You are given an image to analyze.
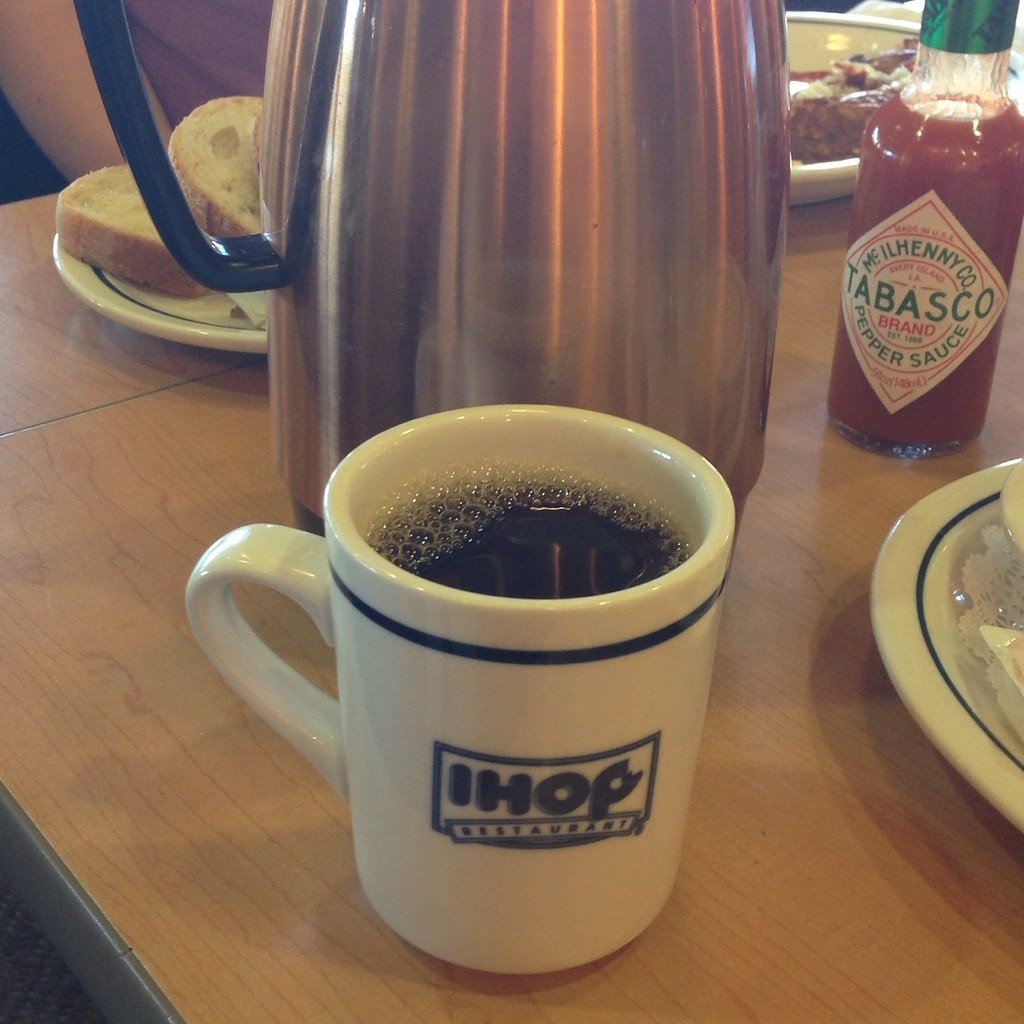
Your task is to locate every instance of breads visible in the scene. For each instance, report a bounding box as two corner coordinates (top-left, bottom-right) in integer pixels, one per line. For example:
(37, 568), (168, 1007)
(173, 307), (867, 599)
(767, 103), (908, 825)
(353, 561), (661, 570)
(56, 94), (263, 300)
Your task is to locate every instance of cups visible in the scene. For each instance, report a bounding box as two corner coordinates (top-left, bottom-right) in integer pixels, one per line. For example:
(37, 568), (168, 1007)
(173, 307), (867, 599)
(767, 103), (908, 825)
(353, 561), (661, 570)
(185, 403), (735, 974)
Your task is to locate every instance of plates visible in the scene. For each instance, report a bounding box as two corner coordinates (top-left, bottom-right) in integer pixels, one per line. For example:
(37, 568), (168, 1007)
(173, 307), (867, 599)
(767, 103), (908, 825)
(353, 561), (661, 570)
(784, 11), (1024, 209)
(868, 456), (1024, 836)
(48, 232), (266, 353)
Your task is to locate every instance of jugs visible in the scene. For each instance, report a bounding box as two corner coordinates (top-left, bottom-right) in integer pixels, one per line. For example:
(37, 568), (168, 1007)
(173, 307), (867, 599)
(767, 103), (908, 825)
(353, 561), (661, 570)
(74, 0), (789, 565)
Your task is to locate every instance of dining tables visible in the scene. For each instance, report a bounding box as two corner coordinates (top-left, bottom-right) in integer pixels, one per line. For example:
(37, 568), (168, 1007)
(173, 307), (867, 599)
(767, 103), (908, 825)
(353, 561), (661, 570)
(0, 0), (1024, 1023)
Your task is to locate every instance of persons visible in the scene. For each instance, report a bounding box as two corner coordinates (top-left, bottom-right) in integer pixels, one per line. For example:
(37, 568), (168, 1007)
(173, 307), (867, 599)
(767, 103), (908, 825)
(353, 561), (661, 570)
(0, 0), (273, 181)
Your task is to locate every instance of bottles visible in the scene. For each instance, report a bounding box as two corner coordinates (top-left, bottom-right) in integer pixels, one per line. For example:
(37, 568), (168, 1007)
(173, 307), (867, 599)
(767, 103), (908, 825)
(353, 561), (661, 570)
(827, 0), (1024, 457)
